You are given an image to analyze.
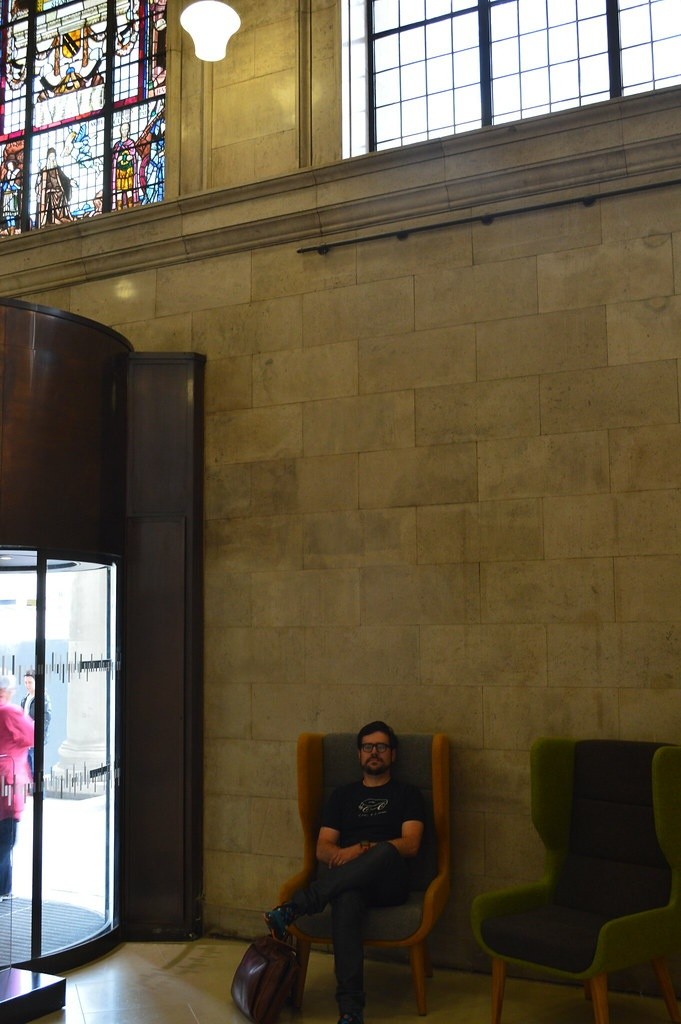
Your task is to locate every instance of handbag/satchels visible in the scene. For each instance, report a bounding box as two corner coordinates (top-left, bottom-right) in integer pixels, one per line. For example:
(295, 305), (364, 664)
(230, 927), (303, 1024)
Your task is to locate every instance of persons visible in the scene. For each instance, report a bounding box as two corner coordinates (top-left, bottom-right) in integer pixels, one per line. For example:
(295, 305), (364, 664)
(261, 721), (428, 1024)
(0, 675), (35, 902)
(19, 671), (52, 800)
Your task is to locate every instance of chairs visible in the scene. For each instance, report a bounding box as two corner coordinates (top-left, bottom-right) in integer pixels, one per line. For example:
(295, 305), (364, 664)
(468, 738), (680, 1024)
(277, 731), (451, 1016)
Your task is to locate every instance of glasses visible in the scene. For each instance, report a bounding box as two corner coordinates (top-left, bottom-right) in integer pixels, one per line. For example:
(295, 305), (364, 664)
(361, 743), (391, 753)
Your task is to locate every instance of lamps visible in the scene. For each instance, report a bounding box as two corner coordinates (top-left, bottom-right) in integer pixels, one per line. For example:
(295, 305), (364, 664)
(179, 0), (241, 62)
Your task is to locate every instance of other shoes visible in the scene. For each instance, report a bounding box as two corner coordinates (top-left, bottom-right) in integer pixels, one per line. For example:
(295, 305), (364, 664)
(0, 893), (16, 903)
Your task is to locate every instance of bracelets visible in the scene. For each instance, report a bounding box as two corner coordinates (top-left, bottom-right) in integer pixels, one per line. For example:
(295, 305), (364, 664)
(360, 841), (371, 852)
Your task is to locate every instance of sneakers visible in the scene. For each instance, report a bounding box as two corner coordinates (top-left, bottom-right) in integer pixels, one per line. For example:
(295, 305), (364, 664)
(262, 901), (299, 929)
(336, 1012), (364, 1024)
